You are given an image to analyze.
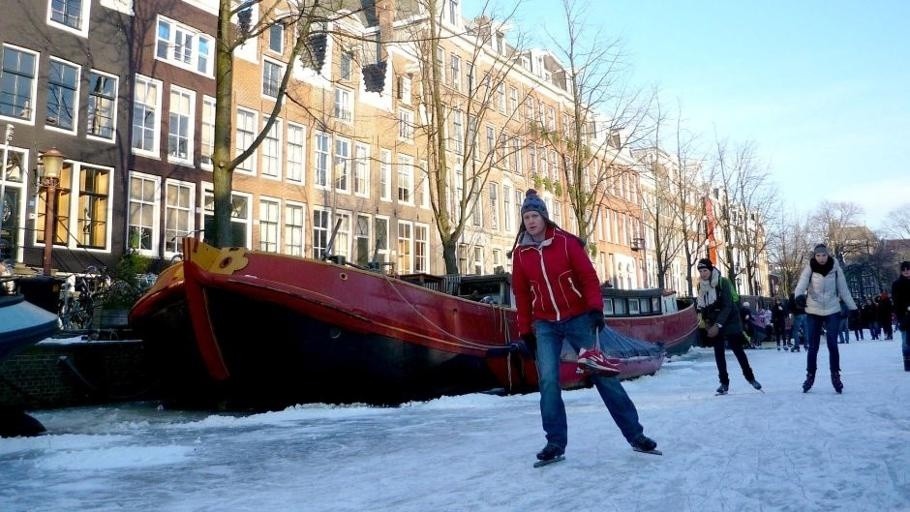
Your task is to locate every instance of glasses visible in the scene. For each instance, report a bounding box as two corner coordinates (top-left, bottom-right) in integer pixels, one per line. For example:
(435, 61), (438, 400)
(901, 267), (910, 271)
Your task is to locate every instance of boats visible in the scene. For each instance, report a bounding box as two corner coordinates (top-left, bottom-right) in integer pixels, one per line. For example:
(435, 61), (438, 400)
(127, 218), (664, 407)
(599, 279), (703, 359)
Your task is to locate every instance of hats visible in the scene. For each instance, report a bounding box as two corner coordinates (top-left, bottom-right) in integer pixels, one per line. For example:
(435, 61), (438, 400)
(697, 258), (713, 271)
(813, 243), (829, 257)
(520, 188), (549, 227)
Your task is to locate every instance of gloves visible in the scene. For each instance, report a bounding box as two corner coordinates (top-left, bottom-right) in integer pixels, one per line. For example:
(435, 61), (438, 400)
(521, 334), (538, 361)
(850, 309), (861, 321)
(590, 310), (605, 334)
(796, 295), (808, 308)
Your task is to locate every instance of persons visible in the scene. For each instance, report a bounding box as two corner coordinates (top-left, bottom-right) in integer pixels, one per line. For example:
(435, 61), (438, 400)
(696, 258), (762, 394)
(794, 242), (859, 391)
(504, 186), (658, 461)
(891, 260), (910, 372)
(692, 289), (893, 348)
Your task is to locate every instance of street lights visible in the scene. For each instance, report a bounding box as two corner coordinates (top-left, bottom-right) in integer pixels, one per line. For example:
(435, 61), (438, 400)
(37, 147), (72, 275)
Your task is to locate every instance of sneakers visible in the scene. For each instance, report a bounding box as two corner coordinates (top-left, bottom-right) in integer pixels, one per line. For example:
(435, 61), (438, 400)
(536, 444), (565, 461)
(716, 384), (728, 392)
(831, 375), (843, 388)
(631, 435), (657, 451)
(802, 374), (815, 389)
(576, 347), (623, 378)
(751, 380), (762, 390)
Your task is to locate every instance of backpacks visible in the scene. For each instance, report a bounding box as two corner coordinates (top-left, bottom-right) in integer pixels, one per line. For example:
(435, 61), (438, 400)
(718, 276), (740, 304)
